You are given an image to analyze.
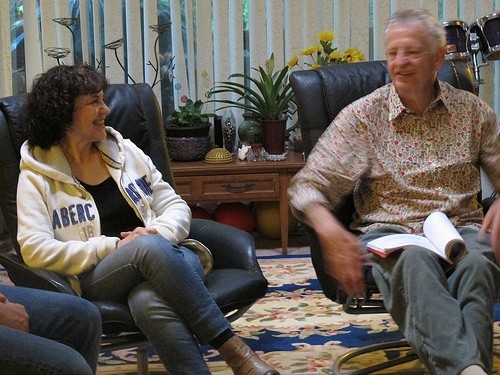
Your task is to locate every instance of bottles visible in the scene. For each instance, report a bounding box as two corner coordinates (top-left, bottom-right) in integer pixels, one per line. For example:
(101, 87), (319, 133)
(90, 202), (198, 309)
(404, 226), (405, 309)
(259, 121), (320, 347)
(238, 110), (262, 146)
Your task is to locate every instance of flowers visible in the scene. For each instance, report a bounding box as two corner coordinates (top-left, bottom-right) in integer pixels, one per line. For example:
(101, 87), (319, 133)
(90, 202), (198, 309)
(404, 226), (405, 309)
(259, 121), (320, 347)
(287, 29), (367, 70)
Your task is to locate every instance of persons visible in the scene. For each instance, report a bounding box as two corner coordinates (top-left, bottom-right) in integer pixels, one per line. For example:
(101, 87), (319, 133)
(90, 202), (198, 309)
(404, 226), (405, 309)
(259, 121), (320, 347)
(16, 65), (280, 375)
(286, 10), (500, 375)
(0, 284), (102, 375)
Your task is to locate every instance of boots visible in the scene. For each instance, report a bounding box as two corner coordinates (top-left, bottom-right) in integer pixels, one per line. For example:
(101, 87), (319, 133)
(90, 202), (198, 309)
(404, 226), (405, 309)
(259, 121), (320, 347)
(216, 334), (280, 375)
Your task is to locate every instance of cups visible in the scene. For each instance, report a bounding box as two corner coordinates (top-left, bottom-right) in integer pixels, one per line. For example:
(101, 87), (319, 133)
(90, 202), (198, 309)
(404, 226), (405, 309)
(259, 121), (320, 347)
(247, 148), (260, 160)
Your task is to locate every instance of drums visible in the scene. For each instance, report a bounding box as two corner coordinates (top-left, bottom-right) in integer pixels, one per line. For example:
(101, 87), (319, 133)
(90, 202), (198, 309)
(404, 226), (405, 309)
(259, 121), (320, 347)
(441, 19), (471, 62)
(471, 10), (500, 60)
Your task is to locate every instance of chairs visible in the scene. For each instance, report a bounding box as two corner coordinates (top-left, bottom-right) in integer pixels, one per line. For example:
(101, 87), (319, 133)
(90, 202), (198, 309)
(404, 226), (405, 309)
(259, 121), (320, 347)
(290, 58), (460, 375)
(0, 82), (270, 375)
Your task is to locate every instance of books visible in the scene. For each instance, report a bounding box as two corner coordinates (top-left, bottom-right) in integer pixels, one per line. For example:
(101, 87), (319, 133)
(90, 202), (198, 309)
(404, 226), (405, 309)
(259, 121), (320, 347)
(366, 212), (467, 273)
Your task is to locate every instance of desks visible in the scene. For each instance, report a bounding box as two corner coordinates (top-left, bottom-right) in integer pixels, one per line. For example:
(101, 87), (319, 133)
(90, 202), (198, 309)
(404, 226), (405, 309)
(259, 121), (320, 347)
(170, 147), (306, 256)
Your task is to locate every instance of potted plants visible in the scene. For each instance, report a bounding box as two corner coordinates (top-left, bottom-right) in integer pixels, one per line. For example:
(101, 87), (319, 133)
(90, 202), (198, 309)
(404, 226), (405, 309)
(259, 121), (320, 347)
(203, 52), (297, 155)
(162, 98), (212, 163)
